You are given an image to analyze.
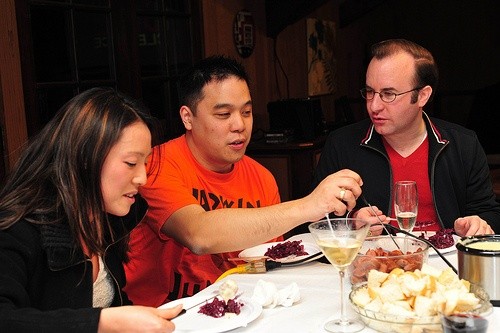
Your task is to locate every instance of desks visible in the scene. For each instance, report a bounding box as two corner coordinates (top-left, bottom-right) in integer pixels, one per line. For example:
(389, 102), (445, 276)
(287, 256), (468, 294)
(197, 233), (500, 333)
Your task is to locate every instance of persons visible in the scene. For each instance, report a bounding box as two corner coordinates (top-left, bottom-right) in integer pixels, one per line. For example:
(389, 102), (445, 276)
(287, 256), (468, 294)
(121, 55), (364, 308)
(0, 87), (184, 333)
(310, 40), (500, 240)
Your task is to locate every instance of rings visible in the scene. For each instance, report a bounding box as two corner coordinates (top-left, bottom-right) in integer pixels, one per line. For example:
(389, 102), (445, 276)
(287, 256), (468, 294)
(367, 231), (372, 237)
(339, 188), (346, 200)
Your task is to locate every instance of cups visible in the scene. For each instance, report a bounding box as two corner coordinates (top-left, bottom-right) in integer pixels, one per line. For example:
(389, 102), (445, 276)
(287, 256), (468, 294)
(438, 301), (494, 333)
(457, 235), (500, 307)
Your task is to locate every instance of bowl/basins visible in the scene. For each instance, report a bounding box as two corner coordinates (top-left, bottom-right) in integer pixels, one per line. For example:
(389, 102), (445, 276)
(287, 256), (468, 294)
(349, 282), (490, 333)
(348, 236), (428, 286)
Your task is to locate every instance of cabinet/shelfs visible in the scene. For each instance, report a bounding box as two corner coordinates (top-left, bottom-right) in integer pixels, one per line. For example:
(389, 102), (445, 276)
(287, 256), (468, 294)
(246, 139), (325, 203)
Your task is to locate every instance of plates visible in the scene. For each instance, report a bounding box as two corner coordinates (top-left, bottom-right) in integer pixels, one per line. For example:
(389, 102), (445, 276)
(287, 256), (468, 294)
(379, 231), (461, 256)
(239, 242), (324, 265)
(156, 296), (260, 333)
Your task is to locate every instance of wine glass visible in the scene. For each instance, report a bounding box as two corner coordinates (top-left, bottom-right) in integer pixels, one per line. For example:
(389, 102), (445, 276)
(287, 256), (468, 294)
(307, 218), (374, 333)
(394, 180), (417, 252)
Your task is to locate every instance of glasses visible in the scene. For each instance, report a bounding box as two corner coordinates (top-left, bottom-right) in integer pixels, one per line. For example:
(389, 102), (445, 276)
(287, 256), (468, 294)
(360, 85), (420, 105)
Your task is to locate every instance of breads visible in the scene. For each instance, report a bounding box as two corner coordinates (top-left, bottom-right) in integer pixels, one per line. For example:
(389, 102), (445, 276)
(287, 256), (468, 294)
(353, 266), (481, 317)
